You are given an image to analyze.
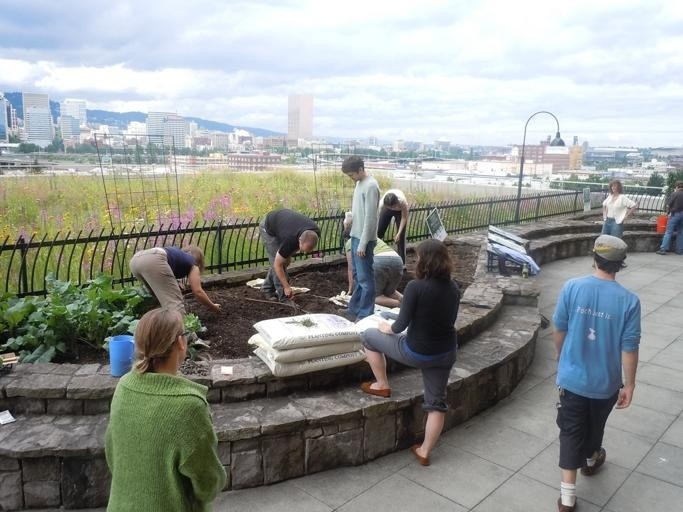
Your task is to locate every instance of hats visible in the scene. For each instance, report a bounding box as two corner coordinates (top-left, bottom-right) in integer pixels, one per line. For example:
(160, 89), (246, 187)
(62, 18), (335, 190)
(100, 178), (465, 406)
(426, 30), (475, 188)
(592, 235), (626, 261)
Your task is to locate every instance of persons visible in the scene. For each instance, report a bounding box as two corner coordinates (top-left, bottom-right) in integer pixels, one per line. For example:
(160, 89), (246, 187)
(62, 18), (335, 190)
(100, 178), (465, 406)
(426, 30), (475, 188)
(102, 307), (228, 511)
(378, 189), (408, 271)
(551, 232), (641, 512)
(601, 180), (637, 268)
(256, 208), (321, 300)
(128, 243), (222, 321)
(655, 180), (683, 255)
(342, 210), (404, 307)
(338, 155), (381, 321)
(356, 238), (460, 468)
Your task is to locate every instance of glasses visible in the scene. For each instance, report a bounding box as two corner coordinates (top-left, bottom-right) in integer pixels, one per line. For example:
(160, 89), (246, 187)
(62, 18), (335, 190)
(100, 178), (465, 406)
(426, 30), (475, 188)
(181, 330), (189, 336)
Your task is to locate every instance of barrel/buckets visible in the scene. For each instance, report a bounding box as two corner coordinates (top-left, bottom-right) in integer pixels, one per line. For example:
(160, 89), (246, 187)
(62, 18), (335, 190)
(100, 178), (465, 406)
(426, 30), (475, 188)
(108, 335), (135, 378)
(656, 215), (668, 234)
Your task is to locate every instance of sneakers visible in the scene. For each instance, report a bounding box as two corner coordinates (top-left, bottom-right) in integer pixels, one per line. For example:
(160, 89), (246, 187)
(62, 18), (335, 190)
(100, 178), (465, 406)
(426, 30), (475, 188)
(557, 496), (576, 512)
(262, 292), (277, 302)
(338, 309), (355, 322)
(360, 383), (390, 397)
(655, 250), (665, 255)
(580, 448), (605, 476)
(411, 445), (429, 466)
(282, 300), (300, 308)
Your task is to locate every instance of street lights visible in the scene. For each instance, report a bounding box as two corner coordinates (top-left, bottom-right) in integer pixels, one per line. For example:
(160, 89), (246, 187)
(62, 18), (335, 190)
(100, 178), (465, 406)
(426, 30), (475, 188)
(513, 110), (566, 224)
